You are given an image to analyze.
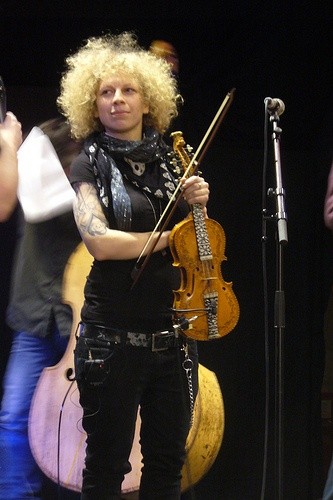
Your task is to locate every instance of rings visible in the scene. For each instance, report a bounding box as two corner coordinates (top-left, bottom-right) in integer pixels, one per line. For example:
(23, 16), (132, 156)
(198, 183), (202, 190)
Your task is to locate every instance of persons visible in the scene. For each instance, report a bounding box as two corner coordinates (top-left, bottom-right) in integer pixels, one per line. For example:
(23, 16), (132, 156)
(0, 111), (23, 222)
(58, 38), (210, 500)
(1, 113), (85, 500)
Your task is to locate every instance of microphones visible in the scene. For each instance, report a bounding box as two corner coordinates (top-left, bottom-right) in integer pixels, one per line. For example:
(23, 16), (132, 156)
(263, 96), (286, 116)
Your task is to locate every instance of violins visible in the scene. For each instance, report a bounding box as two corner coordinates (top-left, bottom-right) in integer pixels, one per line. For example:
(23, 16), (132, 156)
(170, 132), (240, 340)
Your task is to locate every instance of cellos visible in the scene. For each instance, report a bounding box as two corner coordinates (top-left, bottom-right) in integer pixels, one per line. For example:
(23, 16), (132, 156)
(27, 42), (225, 500)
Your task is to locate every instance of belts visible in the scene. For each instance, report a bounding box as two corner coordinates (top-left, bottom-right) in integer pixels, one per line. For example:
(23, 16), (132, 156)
(80, 323), (188, 352)
